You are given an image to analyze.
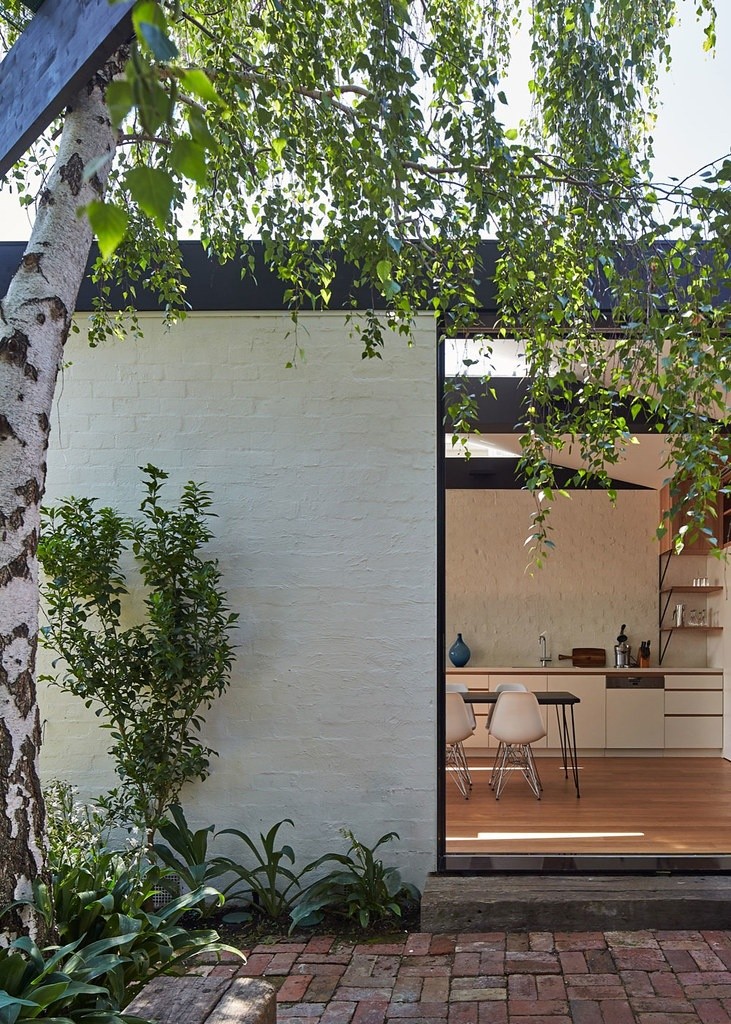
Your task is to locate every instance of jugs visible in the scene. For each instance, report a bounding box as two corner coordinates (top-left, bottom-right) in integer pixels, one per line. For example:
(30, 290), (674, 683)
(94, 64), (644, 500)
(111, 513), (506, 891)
(672, 603), (688, 627)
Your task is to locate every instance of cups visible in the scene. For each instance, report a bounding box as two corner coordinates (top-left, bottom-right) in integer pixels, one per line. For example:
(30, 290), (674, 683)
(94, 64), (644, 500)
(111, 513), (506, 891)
(614, 645), (632, 668)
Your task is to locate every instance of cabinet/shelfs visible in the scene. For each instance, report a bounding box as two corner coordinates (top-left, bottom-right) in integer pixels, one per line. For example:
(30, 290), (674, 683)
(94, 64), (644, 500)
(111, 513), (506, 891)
(664, 668), (723, 757)
(659, 479), (725, 555)
(658, 550), (724, 667)
(445, 667), (488, 758)
(488, 668), (605, 757)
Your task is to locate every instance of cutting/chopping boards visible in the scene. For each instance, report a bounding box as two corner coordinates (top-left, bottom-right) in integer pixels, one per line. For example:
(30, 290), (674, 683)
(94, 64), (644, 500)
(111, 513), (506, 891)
(558, 647), (606, 668)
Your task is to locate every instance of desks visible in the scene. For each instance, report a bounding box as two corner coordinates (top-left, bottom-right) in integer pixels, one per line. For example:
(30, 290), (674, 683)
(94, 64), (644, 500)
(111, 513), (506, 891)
(458, 691), (582, 799)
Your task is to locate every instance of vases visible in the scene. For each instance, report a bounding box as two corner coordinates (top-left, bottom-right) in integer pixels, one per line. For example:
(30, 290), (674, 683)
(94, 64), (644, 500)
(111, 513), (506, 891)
(448, 633), (471, 668)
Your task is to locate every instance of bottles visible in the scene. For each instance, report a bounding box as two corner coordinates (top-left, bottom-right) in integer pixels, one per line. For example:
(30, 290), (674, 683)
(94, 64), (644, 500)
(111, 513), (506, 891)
(449, 632), (471, 667)
(689, 609), (708, 626)
(692, 575), (709, 587)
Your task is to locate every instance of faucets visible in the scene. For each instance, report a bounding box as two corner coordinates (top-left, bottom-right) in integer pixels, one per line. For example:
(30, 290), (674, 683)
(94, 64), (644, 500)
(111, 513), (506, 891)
(537, 635), (553, 667)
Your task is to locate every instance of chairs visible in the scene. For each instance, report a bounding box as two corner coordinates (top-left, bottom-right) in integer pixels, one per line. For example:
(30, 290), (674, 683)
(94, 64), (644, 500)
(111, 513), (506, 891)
(486, 682), (544, 793)
(446, 682), (477, 787)
(446, 691), (476, 800)
(489, 691), (548, 801)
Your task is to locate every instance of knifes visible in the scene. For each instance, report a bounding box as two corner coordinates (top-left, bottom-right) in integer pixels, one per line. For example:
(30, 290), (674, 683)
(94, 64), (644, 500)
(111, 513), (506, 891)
(640, 640), (651, 656)
